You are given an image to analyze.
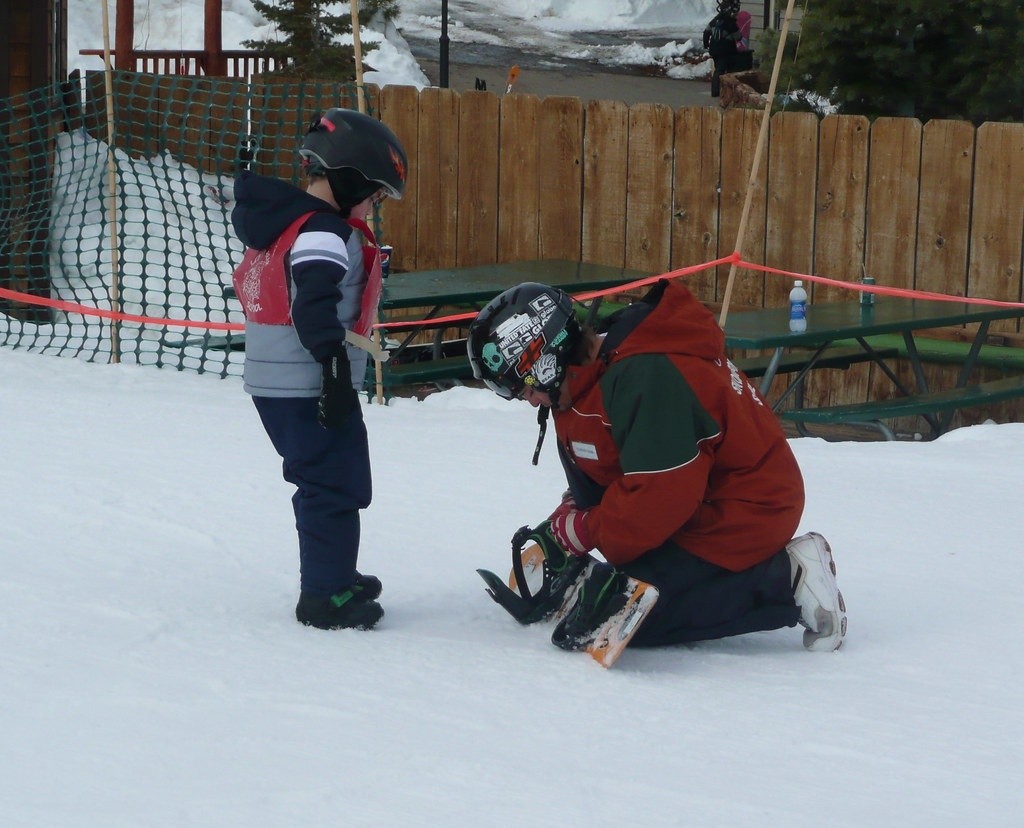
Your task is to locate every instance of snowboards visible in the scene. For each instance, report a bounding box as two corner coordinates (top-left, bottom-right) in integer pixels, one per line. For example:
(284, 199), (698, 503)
(507, 543), (659, 668)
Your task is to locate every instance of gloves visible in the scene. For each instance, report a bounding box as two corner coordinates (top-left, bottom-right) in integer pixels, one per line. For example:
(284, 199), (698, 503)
(547, 494), (578, 522)
(550, 511), (592, 558)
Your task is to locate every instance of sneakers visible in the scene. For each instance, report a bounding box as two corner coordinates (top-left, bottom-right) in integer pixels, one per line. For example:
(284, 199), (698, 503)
(784, 531), (848, 653)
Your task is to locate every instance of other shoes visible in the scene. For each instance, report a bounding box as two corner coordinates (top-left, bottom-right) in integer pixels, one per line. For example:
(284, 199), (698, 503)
(355, 572), (382, 601)
(295, 592), (385, 630)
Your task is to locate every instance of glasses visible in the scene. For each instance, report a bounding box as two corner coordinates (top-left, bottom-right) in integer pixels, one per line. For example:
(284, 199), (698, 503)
(369, 188), (388, 205)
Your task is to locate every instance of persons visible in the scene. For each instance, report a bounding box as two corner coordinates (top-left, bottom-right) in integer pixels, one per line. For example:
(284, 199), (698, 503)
(230, 108), (409, 632)
(466, 284), (848, 671)
(703, 0), (743, 98)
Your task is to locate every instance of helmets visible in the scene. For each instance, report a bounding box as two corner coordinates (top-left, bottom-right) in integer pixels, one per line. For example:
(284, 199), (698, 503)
(465, 281), (574, 401)
(298, 107), (408, 200)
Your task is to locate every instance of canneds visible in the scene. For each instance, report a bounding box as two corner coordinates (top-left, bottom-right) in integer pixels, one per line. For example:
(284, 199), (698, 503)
(859, 277), (874, 305)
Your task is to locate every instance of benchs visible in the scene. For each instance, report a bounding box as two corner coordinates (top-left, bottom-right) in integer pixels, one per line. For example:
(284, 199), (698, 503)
(726, 345), (899, 408)
(370, 357), (473, 392)
(781, 376), (1024, 441)
(388, 310), (473, 335)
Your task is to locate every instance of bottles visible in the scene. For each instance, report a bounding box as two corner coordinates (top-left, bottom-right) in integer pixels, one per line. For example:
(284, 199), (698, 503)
(790, 281), (807, 331)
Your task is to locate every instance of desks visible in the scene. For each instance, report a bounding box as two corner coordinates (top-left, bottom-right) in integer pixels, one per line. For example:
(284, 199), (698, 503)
(383, 258), (658, 366)
(717, 295), (1024, 438)
